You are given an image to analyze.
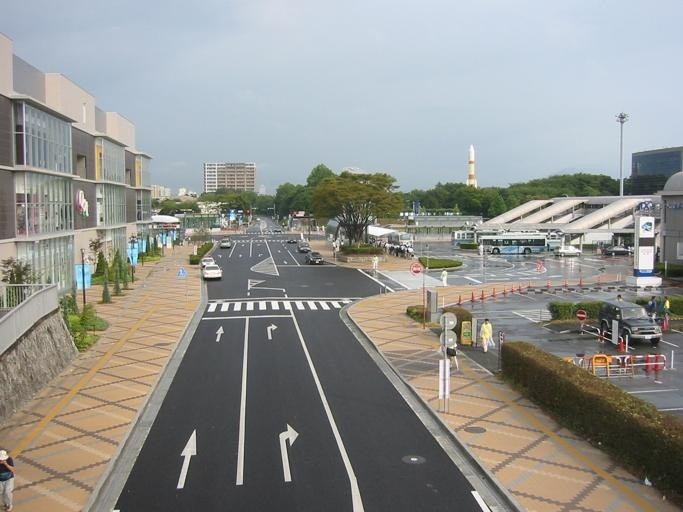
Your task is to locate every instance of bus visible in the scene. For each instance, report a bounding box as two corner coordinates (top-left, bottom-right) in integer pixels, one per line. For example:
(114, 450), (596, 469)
(452, 229), (561, 249)
(477, 234), (548, 255)
(387, 232), (413, 249)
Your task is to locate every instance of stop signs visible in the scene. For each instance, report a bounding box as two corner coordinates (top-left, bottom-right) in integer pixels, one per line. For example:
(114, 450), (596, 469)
(576, 310), (586, 321)
(410, 262), (424, 276)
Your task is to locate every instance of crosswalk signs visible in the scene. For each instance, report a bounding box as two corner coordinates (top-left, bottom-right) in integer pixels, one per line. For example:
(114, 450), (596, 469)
(177, 267), (186, 277)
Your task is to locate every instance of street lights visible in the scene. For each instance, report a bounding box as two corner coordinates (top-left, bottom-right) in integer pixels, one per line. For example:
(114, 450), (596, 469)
(125, 233), (137, 285)
(79, 248), (87, 306)
(615, 110), (630, 196)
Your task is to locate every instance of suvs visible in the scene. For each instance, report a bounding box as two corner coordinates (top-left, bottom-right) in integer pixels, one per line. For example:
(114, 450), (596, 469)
(599, 300), (661, 347)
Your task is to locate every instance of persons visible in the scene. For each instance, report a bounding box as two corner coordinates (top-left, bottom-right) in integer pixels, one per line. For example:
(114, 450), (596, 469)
(614, 295), (670, 321)
(445, 342), (458, 371)
(439, 269), (447, 287)
(478, 319), (492, 352)
(331, 236), (414, 260)
(0, 449), (15, 511)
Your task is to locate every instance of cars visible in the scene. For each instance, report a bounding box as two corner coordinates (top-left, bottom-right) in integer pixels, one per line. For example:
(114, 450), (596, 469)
(304, 251), (323, 265)
(202, 256), (215, 268)
(604, 245), (629, 255)
(298, 240), (311, 252)
(287, 236), (297, 244)
(273, 226), (281, 232)
(202, 264), (222, 279)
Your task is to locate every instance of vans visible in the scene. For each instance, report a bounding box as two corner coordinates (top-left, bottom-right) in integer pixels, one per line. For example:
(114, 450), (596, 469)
(553, 245), (581, 258)
(220, 238), (231, 249)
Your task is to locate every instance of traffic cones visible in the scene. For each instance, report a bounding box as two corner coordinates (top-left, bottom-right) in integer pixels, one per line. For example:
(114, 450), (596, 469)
(660, 318), (667, 331)
(618, 338), (625, 352)
(645, 353), (653, 372)
(654, 354), (662, 371)
